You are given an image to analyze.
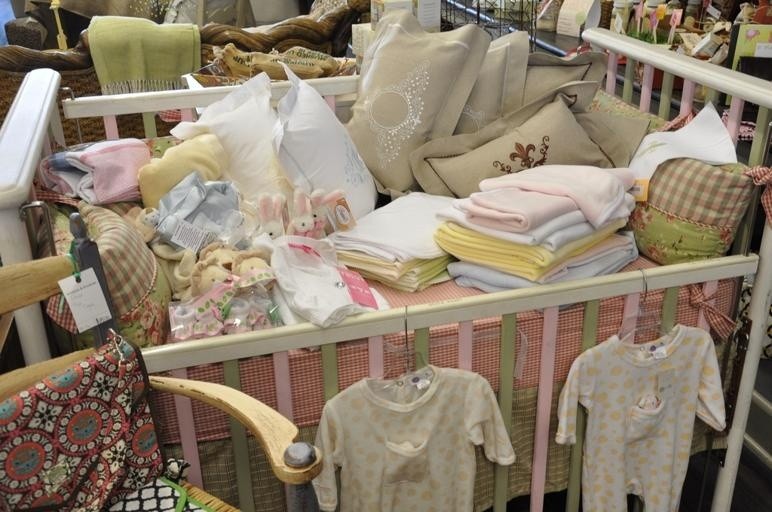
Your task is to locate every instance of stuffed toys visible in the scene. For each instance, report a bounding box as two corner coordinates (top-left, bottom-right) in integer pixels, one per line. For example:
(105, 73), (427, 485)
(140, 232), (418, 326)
(286, 189), (315, 238)
(259, 196), (284, 241)
(124, 206), (188, 262)
(311, 188), (345, 241)
(191, 243), (277, 301)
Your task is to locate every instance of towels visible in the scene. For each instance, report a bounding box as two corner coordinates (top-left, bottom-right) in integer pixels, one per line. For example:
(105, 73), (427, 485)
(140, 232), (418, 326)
(433, 163), (639, 289)
(324, 191), (473, 293)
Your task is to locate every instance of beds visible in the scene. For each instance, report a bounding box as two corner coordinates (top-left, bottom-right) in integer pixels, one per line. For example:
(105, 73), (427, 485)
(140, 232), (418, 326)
(0, 23), (772, 512)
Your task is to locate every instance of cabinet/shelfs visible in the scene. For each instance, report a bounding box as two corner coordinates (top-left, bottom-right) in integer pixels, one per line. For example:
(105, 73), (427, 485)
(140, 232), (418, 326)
(440, 0), (772, 168)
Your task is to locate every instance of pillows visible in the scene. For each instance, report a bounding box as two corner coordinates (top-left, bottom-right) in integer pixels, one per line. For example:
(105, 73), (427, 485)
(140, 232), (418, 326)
(35, 202), (95, 352)
(449, 24), (531, 142)
(623, 162), (756, 265)
(342, 9), (492, 202)
(519, 46), (608, 119)
(74, 192), (171, 354)
(407, 80), (630, 220)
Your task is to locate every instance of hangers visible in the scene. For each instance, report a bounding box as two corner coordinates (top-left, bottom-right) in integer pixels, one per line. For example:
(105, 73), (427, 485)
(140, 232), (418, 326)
(366, 304), (436, 398)
(614, 269), (676, 345)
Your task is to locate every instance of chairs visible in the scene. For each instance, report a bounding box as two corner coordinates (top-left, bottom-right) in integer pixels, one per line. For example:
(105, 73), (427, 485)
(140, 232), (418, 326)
(0, 235), (324, 512)
